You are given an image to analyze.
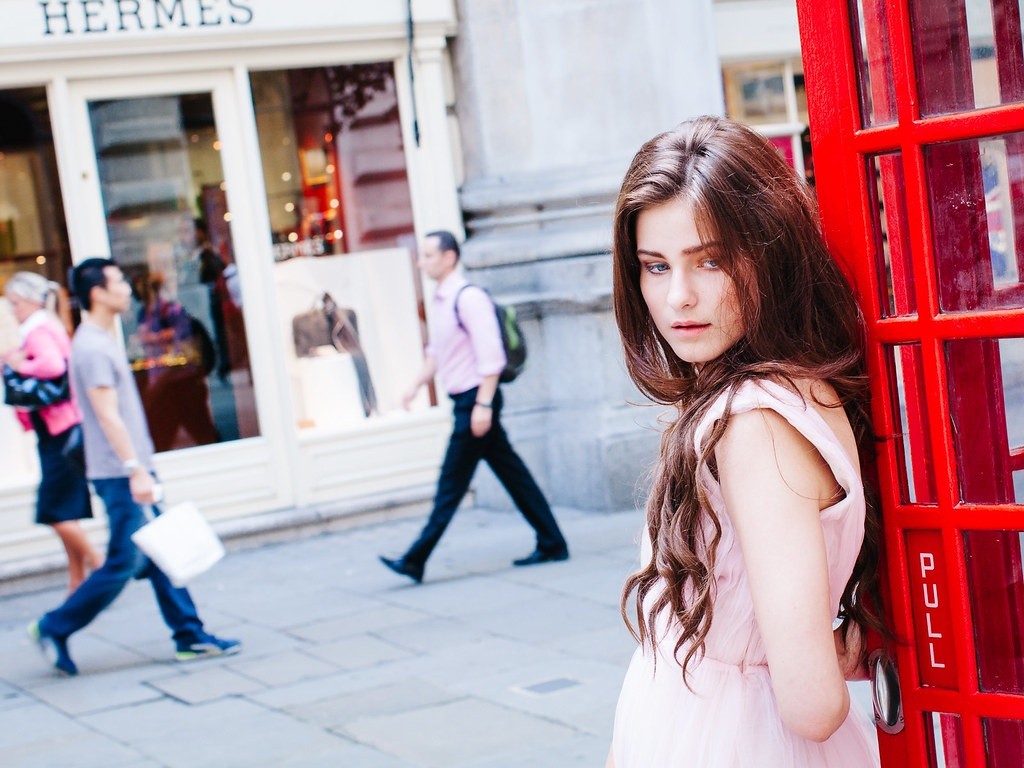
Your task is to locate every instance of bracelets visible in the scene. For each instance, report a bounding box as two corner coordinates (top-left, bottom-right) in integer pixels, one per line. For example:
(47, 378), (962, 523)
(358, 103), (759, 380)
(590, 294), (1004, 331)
(475, 401), (492, 408)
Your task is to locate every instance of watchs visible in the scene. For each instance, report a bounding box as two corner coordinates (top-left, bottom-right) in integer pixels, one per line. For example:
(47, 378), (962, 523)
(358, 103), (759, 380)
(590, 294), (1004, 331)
(121, 459), (140, 476)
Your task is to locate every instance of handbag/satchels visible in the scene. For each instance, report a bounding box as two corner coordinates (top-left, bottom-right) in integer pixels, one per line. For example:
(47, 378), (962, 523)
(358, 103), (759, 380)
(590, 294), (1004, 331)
(131, 498), (226, 588)
(3, 341), (71, 406)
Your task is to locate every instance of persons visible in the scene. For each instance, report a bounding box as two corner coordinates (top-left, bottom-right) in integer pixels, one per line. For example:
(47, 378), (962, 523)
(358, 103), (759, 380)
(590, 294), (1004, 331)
(0, 271), (104, 605)
(115, 262), (230, 453)
(602, 112), (911, 768)
(24, 258), (241, 678)
(378, 230), (570, 584)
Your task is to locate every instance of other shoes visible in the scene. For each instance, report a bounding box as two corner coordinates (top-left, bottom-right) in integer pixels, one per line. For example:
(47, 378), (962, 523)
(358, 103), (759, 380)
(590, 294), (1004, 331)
(513, 550), (569, 565)
(379, 557), (422, 582)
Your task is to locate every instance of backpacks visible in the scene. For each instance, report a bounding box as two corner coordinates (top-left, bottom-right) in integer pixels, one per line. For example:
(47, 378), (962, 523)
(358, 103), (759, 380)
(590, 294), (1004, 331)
(158, 302), (216, 383)
(454, 284), (528, 383)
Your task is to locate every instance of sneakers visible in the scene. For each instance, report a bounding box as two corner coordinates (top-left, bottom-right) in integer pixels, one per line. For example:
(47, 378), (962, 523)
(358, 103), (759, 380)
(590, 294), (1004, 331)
(175, 636), (241, 661)
(28, 620), (78, 677)
